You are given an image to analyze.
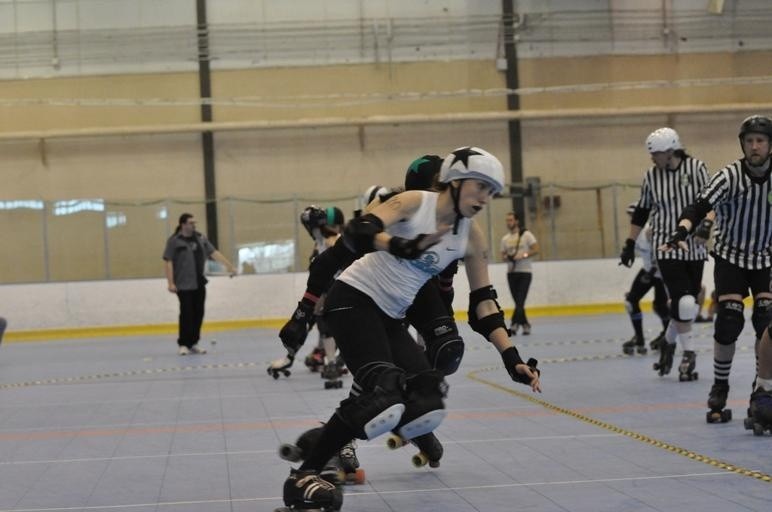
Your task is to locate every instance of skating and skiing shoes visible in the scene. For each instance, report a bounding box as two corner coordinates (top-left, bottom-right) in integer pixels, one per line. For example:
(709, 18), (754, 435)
(678, 350), (699, 381)
(623, 335), (647, 355)
(706, 384), (731, 423)
(386, 429), (443, 467)
(267, 347), (348, 388)
(744, 375), (772, 435)
(278, 421), (366, 512)
(650, 331), (677, 376)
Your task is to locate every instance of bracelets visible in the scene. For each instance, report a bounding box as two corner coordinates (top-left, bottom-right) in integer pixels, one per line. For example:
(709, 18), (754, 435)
(523, 252), (528, 258)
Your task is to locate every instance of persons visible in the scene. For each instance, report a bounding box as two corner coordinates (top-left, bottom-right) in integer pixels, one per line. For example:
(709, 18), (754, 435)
(500, 213), (539, 335)
(624, 201), (672, 347)
(283, 155), (465, 485)
(276, 186), (388, 390)
(282, 147), (543, 511)
(619, 126), (716, 373)
(162, 213), (239, 355)
(655, 112), (772, 424)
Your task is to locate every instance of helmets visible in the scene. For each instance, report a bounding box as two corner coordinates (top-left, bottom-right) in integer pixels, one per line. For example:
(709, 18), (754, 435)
(405, 155), (442, 191)
(364, 185), (388, 204)
(738, 115), (772, 139)
(645, 128), (686, 153)
(321, 206), (343, 228)
(437, 146), (506, 192)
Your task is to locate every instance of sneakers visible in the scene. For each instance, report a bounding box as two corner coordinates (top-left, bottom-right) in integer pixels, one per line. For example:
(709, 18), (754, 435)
(511, 322), (519, 336)
(522, 323), (531, 334)
(178, 345), (206, 355)
